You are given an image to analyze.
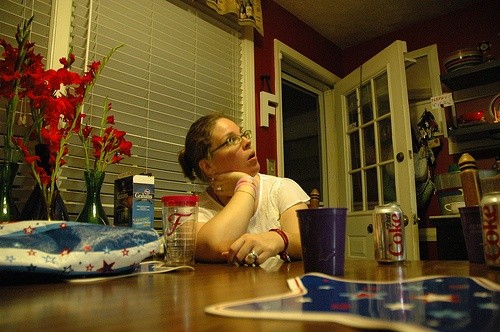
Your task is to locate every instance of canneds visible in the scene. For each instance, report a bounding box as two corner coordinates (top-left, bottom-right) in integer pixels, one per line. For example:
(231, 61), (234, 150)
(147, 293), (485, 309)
(372, 204), (406, 265)
(480, 191), (500, 267)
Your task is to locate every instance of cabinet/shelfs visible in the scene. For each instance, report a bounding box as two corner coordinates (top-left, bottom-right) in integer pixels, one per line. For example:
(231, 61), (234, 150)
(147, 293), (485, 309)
(430, 215), (464, 225)
(439, 62), (500, 156)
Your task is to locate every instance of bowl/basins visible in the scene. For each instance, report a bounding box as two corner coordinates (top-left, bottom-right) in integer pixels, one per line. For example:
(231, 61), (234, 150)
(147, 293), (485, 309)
(443, 48), (482, 74)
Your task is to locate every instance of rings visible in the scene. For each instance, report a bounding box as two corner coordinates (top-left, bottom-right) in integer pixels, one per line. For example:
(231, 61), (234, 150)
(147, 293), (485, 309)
(250, 249), (259, 260)
(216, 185), (223, 192)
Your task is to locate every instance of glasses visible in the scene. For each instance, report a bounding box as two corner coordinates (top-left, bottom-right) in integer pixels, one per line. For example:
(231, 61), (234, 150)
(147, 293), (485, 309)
(209, 130), (252, 154)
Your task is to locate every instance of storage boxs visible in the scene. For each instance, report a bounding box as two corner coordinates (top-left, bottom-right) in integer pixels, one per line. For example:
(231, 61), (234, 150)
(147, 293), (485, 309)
(114, 175), (154, 230)
(433, 169), (499, 216)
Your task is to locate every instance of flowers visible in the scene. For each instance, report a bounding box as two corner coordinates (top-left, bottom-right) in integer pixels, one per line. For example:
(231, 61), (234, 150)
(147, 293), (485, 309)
(0, 15), (66, 162)
(10, 43), (124, 144)
(13, 133), (73, 190)
(76, 95), (133, 173)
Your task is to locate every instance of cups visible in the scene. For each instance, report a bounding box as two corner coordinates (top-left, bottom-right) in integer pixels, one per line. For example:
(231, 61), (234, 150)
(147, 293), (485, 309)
(444, 202), (466, 215)
(296, 208), (347, 277)
(161, 195), (199, 267)
(459, 206), (485, 264)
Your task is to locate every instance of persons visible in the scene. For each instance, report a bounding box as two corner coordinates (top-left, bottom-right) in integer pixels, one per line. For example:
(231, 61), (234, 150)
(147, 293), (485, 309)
(176, 111), (311, 268)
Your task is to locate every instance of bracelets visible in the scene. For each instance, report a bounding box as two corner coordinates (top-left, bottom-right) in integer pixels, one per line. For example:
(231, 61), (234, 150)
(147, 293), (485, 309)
(234, 179), (258, 201)
(267, 228), (292, 263)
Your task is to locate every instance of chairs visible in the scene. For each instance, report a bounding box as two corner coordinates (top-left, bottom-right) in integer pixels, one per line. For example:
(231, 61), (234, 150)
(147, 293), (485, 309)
(461, 170), (500, 207)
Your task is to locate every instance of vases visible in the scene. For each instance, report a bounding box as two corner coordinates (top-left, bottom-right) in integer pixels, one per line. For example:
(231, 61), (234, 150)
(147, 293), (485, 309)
(0, 162), (25, 223)
(73, 171), (109, 225)
(22, 143), (69, 222)
(37, 189), (59, 221)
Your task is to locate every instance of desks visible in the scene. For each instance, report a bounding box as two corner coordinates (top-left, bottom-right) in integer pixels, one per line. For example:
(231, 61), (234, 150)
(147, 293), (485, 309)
(0, 259), (500, 332)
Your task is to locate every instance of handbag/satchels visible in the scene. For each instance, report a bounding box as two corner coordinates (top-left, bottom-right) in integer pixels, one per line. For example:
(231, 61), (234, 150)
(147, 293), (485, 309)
(0, 221), (160, 275)
